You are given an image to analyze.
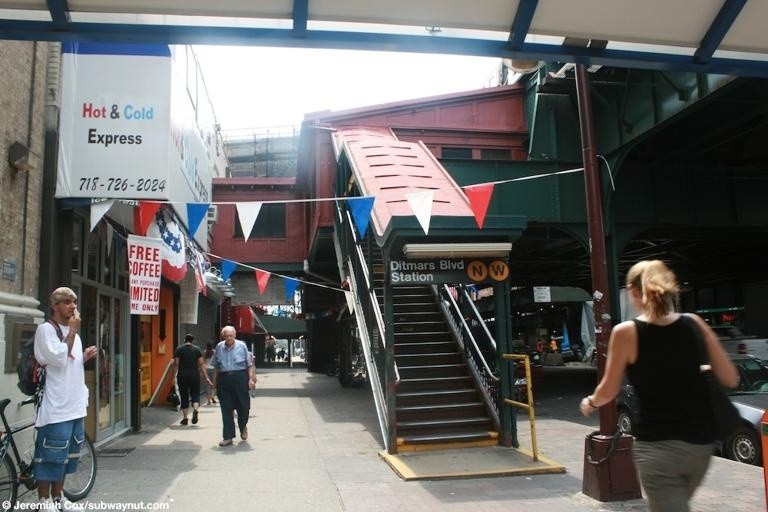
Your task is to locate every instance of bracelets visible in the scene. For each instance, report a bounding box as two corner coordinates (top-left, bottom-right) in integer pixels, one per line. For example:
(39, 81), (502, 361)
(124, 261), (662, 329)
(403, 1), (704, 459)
(587, 395), (599, 410)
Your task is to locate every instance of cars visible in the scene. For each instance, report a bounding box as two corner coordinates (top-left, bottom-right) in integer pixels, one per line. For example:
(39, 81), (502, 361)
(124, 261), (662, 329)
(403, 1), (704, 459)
(614, 352), (768, 467)
(511, 343), (541, 367)
(472, 348), (543, 403)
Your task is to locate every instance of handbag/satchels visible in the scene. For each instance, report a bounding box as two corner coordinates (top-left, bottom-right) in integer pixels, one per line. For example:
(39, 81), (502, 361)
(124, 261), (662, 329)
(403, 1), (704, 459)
(699, 372), (739, 441)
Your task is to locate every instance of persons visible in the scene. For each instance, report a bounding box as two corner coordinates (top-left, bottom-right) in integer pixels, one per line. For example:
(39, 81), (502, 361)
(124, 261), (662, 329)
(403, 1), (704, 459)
(536, 339), (543, 353)
(172, 333), (213, 425)
(202, 340), (217, 405)
(209, 325), (255, 447)
(549, 338), (558, 354)
(31, 286), (99, 512)
(578, 257), (741, 512)
(265, 344), (287, 363)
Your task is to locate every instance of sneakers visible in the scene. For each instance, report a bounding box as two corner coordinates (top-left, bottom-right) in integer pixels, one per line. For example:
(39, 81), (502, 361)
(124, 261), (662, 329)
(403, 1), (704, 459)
(181, 418), (188, 424)
(240, 427), (246, 439)
(192, 409), (198, 424)
(220, 438), (232, 446)
(36, 497), (82, 512)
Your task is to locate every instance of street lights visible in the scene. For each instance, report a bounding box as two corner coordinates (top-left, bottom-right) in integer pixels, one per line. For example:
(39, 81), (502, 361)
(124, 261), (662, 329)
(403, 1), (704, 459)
(502, 53), (645, 502)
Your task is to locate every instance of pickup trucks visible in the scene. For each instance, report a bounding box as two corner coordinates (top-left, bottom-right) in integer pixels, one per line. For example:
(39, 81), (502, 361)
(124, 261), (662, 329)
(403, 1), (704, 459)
(708, 325), (767, 361)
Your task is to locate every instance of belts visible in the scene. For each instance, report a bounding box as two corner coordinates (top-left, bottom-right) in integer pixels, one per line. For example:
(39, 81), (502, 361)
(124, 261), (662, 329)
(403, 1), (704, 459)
(219, 370), (245, 376)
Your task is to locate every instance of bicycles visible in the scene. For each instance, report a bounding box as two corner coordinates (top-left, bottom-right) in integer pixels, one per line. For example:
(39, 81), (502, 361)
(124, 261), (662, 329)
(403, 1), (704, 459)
(0, 395), (98, 511)
(324, 358), (342, 377)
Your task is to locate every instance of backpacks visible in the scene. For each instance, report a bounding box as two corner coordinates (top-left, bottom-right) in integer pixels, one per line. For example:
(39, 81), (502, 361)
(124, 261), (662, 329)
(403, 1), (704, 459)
(17, 319), (63, 396)
